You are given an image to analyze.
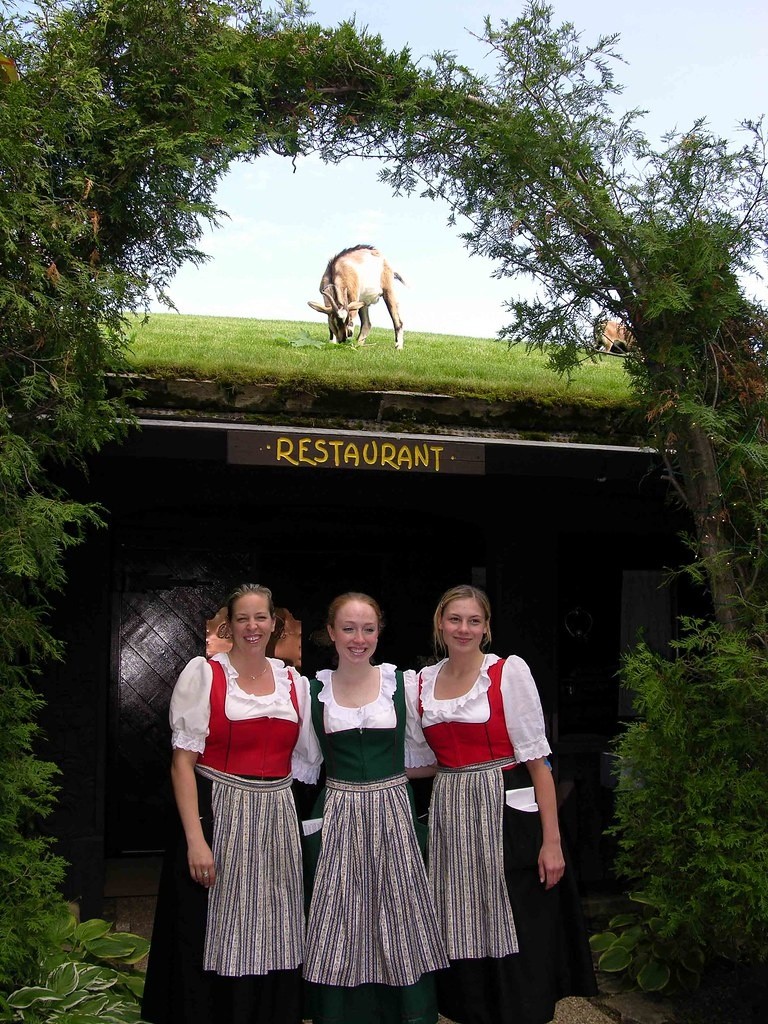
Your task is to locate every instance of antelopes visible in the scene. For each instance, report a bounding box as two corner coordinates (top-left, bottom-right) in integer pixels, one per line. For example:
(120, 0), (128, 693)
(307, 244), (408, 350)
(594, 320), (637, 355)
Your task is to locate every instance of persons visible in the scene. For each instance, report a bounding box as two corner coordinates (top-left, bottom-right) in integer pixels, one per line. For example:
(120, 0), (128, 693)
(143, 584), (600, 1024)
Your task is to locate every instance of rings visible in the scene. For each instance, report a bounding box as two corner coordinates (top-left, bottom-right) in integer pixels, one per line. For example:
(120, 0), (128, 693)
(203, 872), (208, 878)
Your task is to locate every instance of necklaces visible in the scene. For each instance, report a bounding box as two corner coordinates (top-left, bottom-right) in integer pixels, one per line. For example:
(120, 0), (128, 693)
(238, 657), (268, 680)
